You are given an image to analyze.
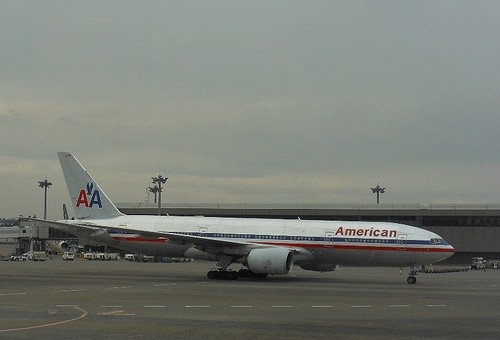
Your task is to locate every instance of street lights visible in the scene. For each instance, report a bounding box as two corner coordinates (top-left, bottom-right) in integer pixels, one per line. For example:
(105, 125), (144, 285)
(371, 181), (386, 203)
(38, 176), (52, 218)
(148, 172), (168, 209)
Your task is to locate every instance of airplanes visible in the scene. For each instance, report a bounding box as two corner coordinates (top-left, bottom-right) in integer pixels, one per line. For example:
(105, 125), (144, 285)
(26, 150), (454, 285)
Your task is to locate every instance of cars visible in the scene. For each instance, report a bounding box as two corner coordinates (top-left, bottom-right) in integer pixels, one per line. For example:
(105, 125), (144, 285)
(12, 250), (45, 262)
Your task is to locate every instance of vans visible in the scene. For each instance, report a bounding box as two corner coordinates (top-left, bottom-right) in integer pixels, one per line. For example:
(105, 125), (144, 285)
(61, 252), (74, 260)
